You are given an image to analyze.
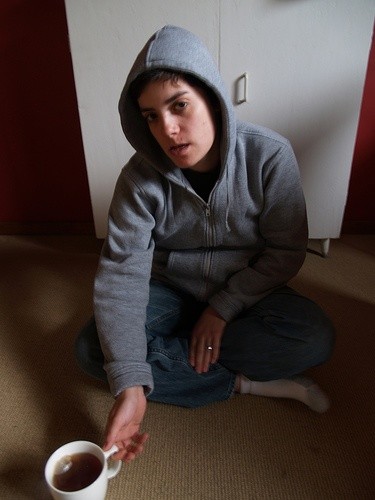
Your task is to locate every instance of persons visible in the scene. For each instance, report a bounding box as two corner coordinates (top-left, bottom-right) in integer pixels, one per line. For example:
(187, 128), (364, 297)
(70, 23), (339, 465)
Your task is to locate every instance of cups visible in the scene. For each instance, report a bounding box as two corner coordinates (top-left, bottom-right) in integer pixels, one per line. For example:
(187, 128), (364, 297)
(44, 441), (122, 500)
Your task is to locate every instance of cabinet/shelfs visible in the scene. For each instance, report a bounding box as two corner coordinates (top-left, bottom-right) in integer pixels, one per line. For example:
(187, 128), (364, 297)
(65, 0), (374, 256)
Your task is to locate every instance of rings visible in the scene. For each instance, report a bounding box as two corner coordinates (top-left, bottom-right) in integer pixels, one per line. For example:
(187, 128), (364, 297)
(205, 345), (213, 350)
